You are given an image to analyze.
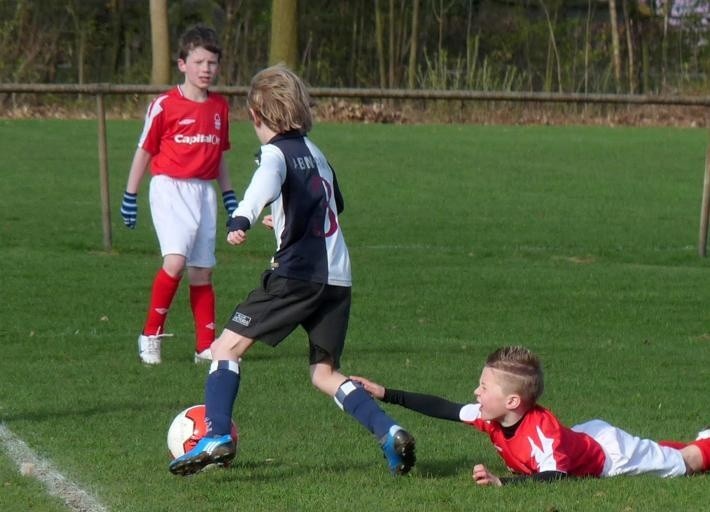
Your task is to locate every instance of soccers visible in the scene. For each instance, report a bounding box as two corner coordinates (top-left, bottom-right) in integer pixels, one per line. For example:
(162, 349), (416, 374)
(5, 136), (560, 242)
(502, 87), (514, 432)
(166, 405), (238, 471)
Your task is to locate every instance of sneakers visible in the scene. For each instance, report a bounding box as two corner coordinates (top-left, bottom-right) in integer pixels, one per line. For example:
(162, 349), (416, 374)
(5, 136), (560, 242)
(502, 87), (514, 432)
(195, 348), (212, 363)
(169, 434), (235, 477)
(138, 334), (162, 364)
(382, 424), (416, 474)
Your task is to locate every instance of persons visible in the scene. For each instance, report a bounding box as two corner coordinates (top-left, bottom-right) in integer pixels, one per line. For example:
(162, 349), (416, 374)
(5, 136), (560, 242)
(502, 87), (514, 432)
(348, 345), (710, 487)
(119, 25), (243, 369)
(168, 66), (416, 476)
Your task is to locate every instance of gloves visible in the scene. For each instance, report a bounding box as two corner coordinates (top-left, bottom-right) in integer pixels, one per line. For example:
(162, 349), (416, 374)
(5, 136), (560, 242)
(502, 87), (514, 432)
(222, 190), (237, 217)
(121, 191), (137, 229)
